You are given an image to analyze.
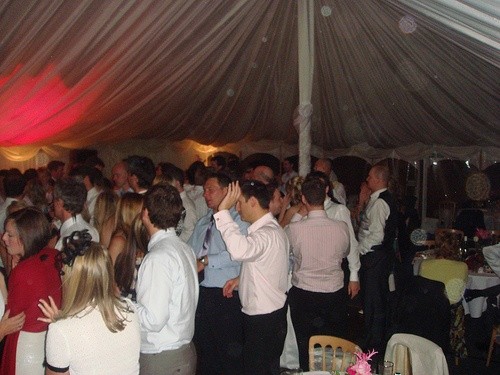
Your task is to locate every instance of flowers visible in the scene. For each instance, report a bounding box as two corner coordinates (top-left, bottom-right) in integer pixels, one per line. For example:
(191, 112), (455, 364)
(477, 228), (492, 239)
(347, 346), (378, 375)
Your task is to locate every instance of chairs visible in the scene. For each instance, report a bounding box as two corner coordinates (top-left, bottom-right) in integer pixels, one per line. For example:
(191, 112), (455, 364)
(435, 228), (465, 248)
(384, 334), (450, 375)
(395, 259), (500, 366)
(309, 335), (362, 373)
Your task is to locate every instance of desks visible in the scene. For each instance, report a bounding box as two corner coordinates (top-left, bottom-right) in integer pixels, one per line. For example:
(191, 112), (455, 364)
(412, 260), (500, 318)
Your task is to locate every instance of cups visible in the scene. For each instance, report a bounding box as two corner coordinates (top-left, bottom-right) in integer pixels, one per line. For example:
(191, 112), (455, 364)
(375, 360), (394, 375)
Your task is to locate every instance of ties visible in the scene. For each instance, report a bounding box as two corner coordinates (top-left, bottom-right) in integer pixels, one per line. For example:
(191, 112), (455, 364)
(201, 213), (218, 255)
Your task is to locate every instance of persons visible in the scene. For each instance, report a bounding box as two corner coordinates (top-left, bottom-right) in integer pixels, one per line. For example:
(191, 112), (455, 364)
(358, 165), (396, 365)
(1, 154), (359, 375)
(448, 196), (484, 238)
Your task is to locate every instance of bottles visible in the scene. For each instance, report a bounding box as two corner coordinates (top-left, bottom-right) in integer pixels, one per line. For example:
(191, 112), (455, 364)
(454, 234), (481, 270)
(426, 232), (436, 259)
